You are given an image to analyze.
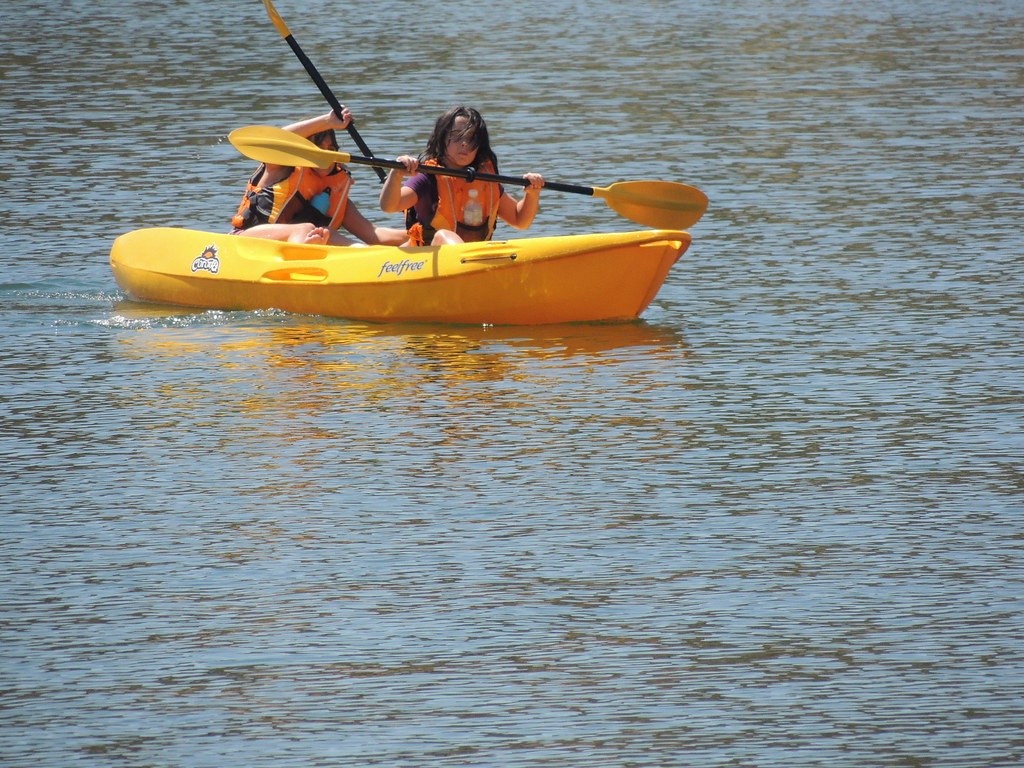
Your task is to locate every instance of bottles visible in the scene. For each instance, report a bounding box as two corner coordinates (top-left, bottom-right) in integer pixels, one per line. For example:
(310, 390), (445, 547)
(298, 187), (331, 226)
(463, 189), (482, 242)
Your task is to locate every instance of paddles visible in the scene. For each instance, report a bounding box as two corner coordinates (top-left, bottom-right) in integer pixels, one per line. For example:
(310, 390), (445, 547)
(224, 123), (711, 230)
(261, 2), (408, 216)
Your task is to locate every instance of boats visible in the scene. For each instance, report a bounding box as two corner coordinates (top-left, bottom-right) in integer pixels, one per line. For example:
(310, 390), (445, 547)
(109, 226), (692, 328)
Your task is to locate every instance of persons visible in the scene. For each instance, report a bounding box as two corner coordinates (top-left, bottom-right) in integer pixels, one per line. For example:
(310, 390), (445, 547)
(228, 103), (409, 246)
(379, 106), (545, 245)
